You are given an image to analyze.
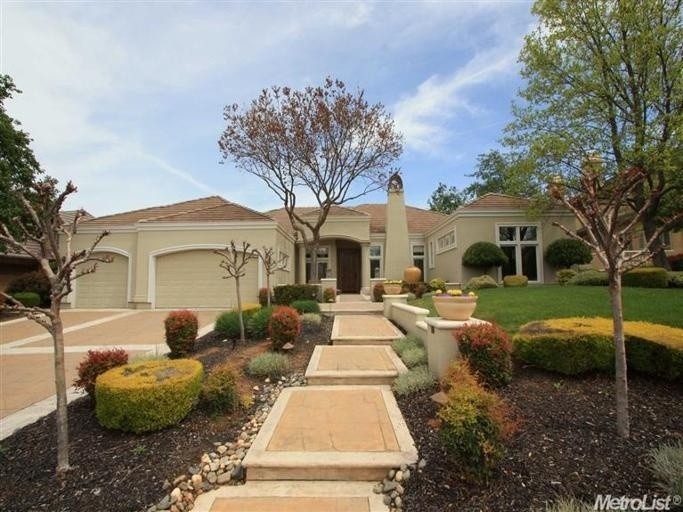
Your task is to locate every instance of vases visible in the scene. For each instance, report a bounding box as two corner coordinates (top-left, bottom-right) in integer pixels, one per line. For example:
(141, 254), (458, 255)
(384, 285), (402, 295)
(433, 296), (477, 322)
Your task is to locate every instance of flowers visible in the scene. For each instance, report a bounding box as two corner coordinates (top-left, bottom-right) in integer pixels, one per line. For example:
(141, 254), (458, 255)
(383, 279), (402, 284)
(435, 287), (476, 297)
